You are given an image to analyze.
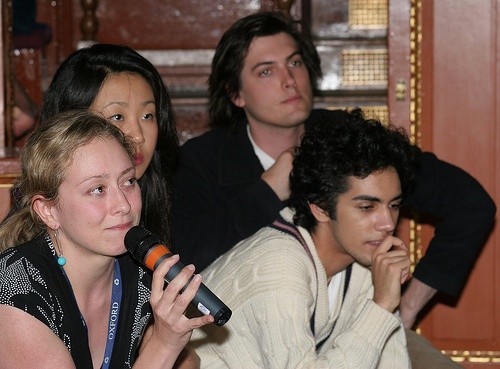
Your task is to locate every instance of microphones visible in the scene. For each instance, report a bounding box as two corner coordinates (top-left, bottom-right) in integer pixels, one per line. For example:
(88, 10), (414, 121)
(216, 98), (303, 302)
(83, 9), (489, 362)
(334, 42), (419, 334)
(124, 225), (232, 326)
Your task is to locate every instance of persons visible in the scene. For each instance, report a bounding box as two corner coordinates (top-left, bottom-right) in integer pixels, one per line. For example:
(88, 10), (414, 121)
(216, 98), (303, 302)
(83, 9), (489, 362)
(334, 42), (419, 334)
(0, 11), (497, 369)
